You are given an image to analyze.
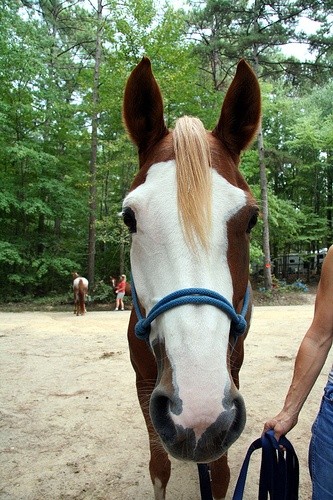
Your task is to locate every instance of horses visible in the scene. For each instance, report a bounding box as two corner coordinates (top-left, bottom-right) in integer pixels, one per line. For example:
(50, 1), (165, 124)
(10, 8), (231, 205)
(109, 276), (132, 310)
(120, 56), (262, 500)
(72, 272), (89, 316)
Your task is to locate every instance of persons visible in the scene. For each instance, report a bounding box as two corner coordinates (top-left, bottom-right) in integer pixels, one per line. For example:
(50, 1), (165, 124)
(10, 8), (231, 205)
(112, 274), (126, 312)
(261, 244), (333, 500)
